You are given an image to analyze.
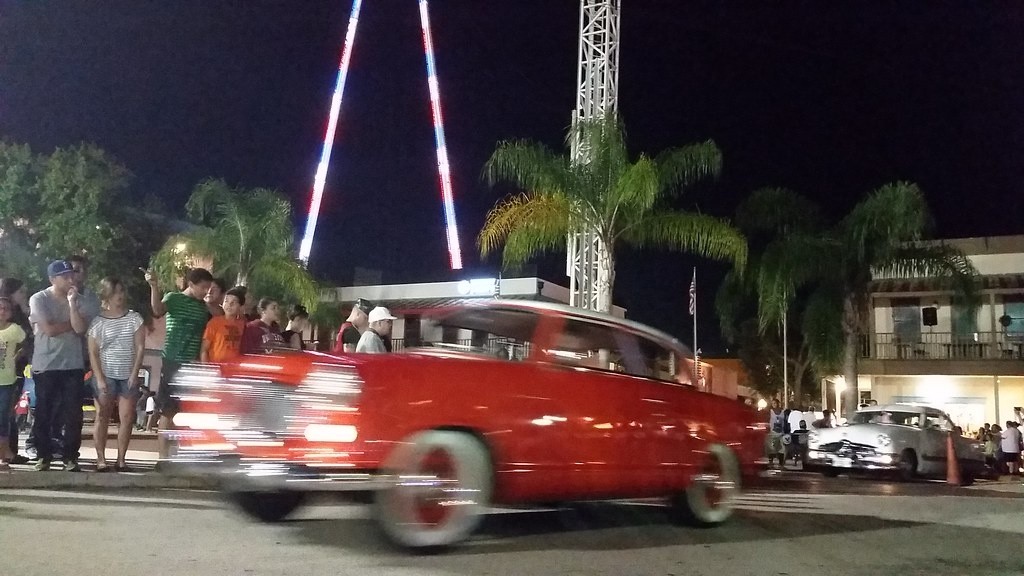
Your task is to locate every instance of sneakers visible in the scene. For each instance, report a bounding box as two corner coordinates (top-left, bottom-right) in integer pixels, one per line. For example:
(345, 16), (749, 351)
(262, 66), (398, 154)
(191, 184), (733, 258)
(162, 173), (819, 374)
(35, 457), (51, 470)
(64, 460), (81, 472)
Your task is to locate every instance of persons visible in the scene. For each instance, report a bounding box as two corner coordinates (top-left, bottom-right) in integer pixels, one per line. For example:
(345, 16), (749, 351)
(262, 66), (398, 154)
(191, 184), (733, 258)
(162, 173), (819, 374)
(0, 256), (396, 472)
(977, 407), (1024, 475)
(766, 399), (833, 470)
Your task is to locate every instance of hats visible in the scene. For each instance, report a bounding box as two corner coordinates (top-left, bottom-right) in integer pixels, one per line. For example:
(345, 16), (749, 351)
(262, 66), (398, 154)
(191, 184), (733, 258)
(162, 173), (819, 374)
(355, 298), (373, 316)
(47, 259), (80, 277)
(368, 306), (397, 323)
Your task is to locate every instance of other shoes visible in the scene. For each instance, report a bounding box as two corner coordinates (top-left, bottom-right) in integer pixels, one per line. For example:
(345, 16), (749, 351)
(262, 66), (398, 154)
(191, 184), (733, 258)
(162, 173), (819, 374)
(52, 447), (80, 459)
(27, 447), (38, 460)
(3, 454), (29, 464)
(96, 463), (110, 473)
(115, 463), (133, 472)
(154, 461), (170, 473)
(778, 466), (787, 470)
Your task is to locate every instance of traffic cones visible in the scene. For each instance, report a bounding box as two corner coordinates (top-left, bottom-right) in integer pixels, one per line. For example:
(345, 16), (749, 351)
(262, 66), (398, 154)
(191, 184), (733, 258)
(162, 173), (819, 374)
(945, 431), (967, 486)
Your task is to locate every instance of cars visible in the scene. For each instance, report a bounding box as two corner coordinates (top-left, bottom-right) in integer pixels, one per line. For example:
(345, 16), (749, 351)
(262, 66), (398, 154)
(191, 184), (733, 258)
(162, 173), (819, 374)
(150, 298), (770, 557)
(808, 403), (983, 486)
